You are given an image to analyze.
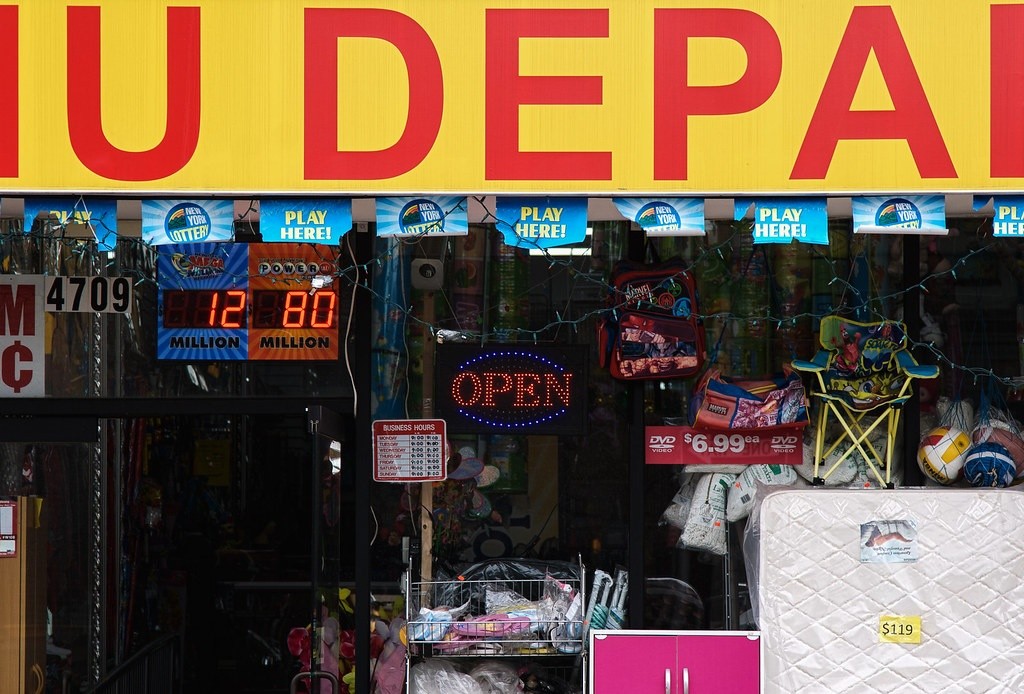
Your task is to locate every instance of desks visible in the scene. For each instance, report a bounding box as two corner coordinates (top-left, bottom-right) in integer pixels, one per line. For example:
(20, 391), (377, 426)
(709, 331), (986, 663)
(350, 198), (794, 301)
(216, 578), (403, 594)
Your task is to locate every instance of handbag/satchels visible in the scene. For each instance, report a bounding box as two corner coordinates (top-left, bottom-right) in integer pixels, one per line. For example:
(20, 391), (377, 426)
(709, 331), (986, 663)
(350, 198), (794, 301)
(687, 367), (811, 430)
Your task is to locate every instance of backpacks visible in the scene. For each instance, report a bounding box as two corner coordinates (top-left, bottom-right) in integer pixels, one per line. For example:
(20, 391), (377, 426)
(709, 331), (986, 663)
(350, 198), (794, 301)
(597, 239), (708, 380)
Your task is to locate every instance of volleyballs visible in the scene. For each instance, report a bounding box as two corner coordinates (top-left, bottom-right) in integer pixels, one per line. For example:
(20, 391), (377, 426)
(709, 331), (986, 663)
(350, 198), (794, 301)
(963, 441), (1017, 489)
(916, 425), (971, 486)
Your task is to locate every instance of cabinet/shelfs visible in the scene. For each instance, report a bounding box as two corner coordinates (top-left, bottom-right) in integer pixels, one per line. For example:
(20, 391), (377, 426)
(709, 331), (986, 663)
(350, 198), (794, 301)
(589, 628), (765, 694)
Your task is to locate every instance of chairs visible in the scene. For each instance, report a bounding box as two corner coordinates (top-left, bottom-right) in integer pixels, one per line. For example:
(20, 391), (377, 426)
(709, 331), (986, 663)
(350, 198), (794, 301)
(790, 315), (940, 491)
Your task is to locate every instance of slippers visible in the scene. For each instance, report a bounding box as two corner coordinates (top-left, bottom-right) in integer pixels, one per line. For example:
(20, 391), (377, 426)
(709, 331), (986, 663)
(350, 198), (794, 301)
(412, 612), (531, 652)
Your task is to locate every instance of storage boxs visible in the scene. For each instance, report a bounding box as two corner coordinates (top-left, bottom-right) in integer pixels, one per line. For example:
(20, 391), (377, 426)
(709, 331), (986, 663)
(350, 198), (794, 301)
(645, 424), (805, 465)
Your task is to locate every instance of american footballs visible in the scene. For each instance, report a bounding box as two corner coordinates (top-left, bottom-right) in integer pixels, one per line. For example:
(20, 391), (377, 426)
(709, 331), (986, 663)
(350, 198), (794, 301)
(972, 427), (1024, 479)
